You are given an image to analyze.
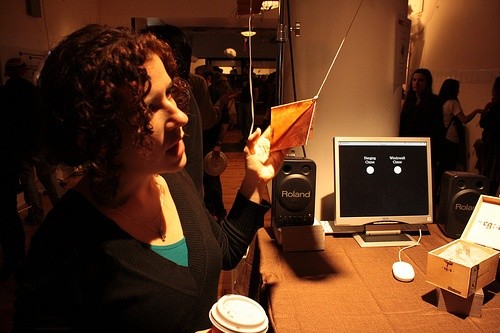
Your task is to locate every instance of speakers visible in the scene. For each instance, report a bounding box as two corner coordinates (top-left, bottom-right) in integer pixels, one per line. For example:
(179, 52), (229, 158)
(271, 158), (317, 226)
(26, 0), (42, 17)
(435, 172), (491, 240)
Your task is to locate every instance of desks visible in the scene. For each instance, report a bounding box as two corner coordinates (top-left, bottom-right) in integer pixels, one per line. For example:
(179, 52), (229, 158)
(248, 219), (500, 332)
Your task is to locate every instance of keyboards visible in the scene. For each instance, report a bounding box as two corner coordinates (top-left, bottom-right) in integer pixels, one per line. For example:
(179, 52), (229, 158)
(330, 225), (429, 232)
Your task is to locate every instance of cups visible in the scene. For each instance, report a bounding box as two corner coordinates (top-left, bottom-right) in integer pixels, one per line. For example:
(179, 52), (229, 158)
(208, 294), (269, 333)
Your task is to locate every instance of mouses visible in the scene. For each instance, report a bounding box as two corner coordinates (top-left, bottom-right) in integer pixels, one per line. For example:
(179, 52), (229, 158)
(393, 261), (415, 281)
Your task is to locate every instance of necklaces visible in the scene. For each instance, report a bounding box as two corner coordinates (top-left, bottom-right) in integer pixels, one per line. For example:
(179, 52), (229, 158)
(97, 173), (167, 243)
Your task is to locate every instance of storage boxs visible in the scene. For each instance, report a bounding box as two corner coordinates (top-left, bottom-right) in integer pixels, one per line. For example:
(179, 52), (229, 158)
(425, 195), (500, 299)
(435, 285), (484, 318)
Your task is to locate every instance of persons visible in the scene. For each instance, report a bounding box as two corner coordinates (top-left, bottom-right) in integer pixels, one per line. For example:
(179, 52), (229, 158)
(12, 24), (294, 333)
(0, 58), (83, 278)
(142, 21), (277, 227)
(399, 69), (500, 200)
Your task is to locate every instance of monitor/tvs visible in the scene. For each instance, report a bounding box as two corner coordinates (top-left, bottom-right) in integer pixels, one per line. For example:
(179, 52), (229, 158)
(333, 137), (432, 225)
